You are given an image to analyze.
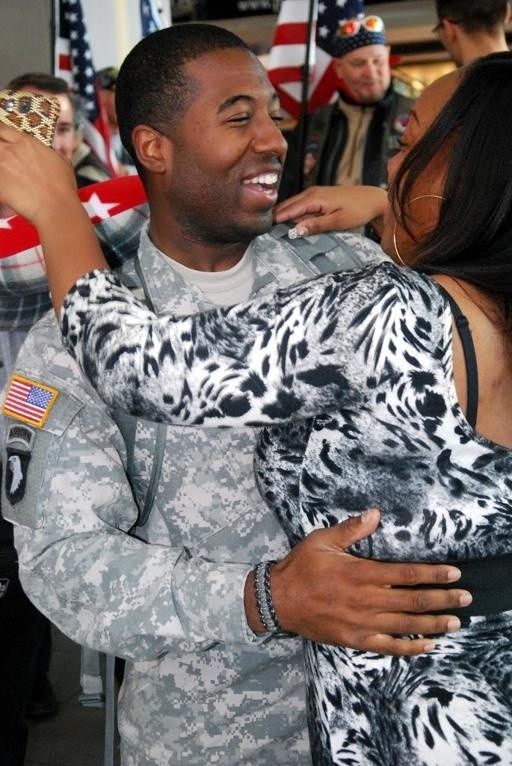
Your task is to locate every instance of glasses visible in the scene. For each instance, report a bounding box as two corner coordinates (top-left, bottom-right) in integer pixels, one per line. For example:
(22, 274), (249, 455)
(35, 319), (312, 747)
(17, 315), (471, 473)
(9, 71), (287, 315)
(336, 15), (386, 36)
(430, 18), (461, 43)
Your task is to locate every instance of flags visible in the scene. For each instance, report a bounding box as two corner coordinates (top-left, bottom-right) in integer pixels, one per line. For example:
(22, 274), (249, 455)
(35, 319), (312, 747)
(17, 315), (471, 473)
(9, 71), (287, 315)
(267, 1), (339, 119)
(48, 0), (114, 176)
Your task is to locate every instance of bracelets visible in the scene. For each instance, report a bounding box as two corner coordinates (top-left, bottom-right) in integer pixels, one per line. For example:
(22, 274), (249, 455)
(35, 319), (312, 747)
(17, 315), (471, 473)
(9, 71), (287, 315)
(254, 560), (281, 633)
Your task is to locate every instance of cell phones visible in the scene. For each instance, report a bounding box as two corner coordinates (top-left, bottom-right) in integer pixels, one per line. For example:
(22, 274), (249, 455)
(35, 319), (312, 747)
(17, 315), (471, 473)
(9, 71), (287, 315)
(0, 90), (60, 149)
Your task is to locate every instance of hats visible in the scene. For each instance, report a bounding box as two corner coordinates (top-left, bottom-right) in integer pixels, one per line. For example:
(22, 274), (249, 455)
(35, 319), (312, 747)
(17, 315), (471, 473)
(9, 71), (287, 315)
(327, 15), (388, 56)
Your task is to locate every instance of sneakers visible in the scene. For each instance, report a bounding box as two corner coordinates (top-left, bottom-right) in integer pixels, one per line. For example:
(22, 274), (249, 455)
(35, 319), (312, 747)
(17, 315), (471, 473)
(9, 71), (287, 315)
(26, 675), (59, 720)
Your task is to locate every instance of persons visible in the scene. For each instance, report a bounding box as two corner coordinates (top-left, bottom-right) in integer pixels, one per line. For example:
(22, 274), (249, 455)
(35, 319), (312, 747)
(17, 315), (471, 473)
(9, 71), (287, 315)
(301, 13), (418, 244)
(3, 22), (473, 765)
(1, 47), (511, 765)
(432, 1), (510, 68)
(3, 72), (74, 161)
